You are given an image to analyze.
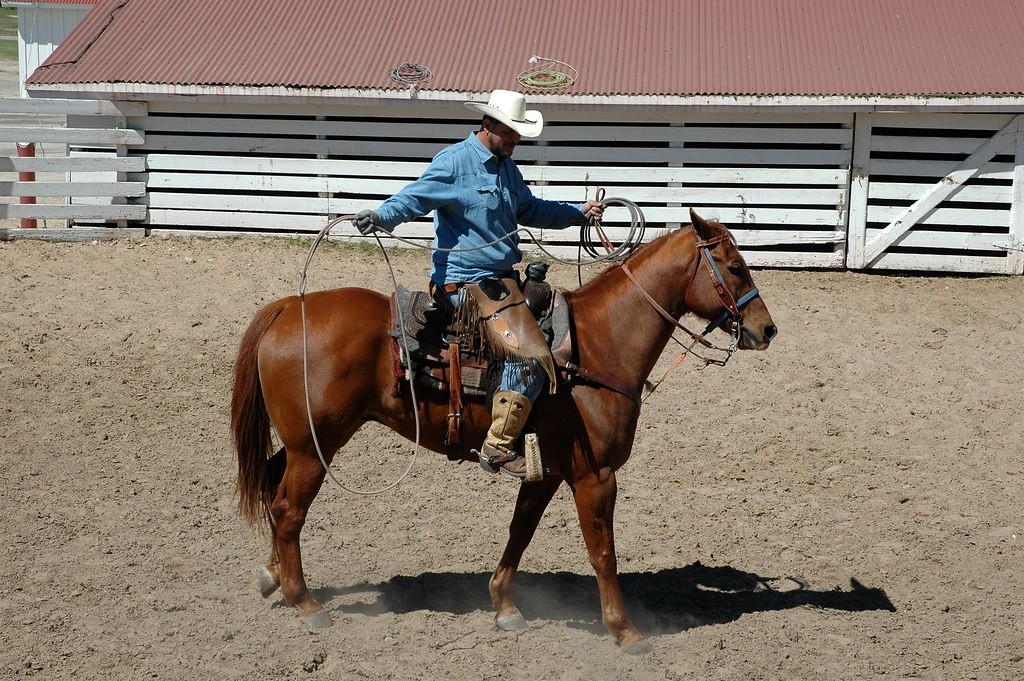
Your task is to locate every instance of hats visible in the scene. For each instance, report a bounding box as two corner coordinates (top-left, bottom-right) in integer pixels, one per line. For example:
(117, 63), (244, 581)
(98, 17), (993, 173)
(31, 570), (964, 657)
(463, 89), (544, 138)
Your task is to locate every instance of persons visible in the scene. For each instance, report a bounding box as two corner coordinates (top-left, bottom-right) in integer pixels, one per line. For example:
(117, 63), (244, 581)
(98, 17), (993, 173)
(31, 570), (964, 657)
(350, 90), (608, 476)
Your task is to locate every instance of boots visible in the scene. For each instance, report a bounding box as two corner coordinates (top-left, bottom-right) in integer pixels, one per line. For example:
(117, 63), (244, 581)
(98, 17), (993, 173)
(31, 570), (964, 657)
(478, 389), (528, 477)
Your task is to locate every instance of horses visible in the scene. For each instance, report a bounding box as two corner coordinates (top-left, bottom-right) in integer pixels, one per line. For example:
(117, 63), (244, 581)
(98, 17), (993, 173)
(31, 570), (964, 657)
(230, 205), (779, 656)
